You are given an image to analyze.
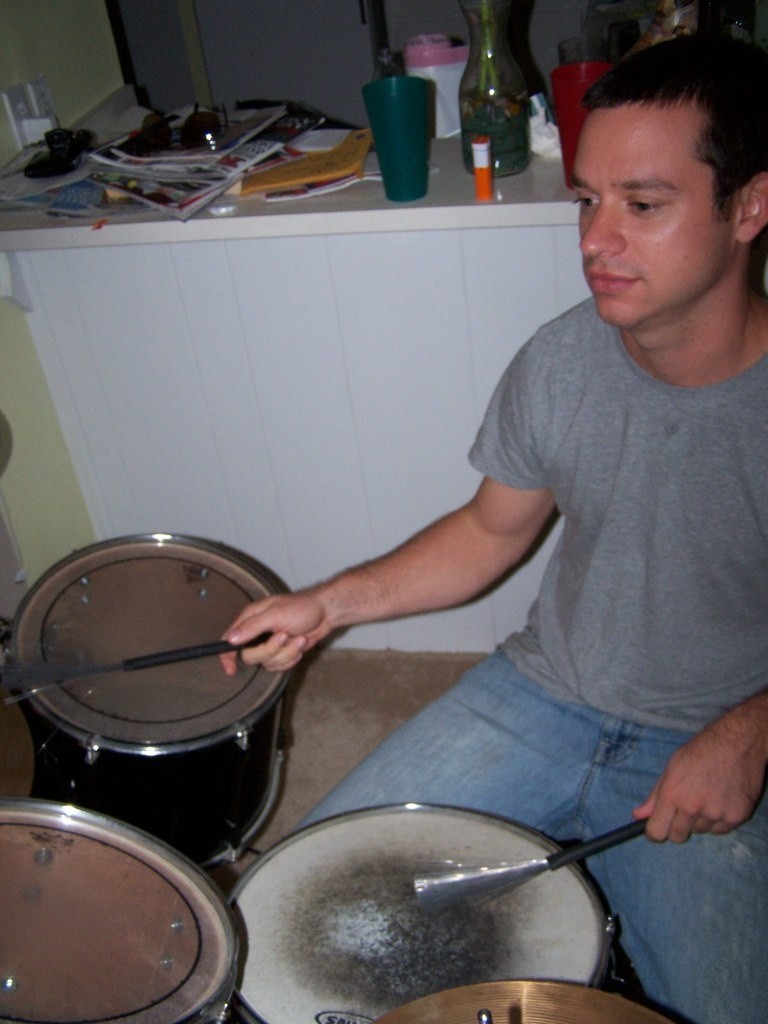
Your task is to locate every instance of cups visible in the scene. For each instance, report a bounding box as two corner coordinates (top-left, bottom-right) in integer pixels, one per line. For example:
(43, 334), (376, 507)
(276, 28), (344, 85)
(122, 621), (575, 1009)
(363, 76), (435, 202)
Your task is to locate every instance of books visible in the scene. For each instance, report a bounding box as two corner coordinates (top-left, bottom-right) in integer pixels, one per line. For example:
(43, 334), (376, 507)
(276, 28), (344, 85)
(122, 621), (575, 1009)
(87, 105), (302, 223)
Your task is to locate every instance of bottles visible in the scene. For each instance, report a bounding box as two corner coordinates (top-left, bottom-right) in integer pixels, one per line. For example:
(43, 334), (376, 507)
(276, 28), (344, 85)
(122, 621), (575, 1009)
(375, 49), (407, 77)
(551, 64), (616, 187)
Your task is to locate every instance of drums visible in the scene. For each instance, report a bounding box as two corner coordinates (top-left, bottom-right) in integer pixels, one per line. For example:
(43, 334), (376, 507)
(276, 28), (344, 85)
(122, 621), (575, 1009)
(13, 533), (292, 874)
(1, 791), (245, 1024)
(227, 799), (614, 1024)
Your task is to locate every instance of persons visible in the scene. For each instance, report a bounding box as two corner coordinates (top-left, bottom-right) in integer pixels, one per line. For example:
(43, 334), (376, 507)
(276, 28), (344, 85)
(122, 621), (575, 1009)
(218, 34), (768, 1024)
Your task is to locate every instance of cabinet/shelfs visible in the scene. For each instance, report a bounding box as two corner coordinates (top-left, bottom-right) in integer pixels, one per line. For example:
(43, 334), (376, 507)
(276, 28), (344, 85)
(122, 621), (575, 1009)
(0, 131), (592, 653)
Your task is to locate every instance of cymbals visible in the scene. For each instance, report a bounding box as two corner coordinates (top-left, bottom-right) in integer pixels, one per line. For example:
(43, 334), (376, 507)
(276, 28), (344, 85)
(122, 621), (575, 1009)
(369, 976), (675, 1024)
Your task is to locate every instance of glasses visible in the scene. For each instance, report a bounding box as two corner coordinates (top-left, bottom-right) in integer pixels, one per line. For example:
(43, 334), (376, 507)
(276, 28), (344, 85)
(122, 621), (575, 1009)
(138, 102), (228, 153)
